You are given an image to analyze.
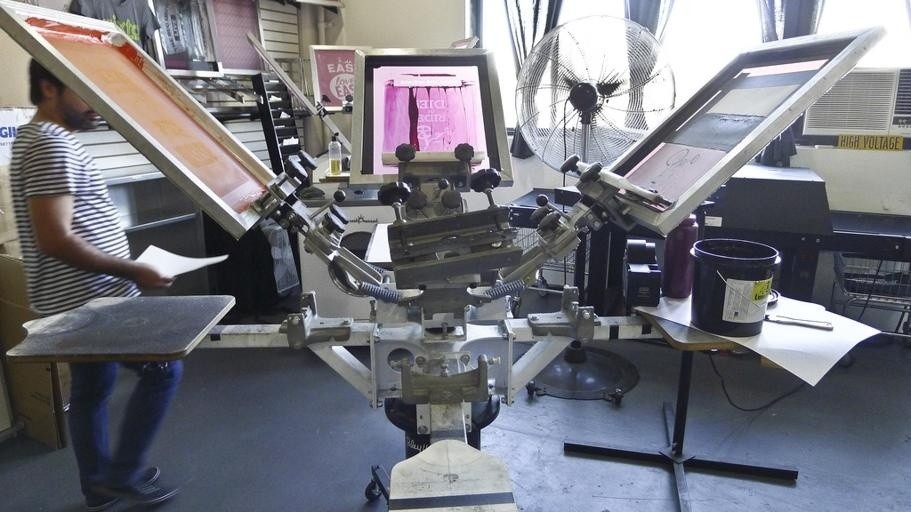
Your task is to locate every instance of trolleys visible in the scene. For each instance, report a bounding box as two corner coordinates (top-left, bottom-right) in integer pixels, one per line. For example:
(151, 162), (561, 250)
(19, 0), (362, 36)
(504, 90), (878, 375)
(827, 250), (911, 367)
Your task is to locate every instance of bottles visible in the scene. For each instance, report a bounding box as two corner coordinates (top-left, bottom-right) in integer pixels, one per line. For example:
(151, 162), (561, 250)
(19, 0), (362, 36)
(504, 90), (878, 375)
(328, 135), (342, 176)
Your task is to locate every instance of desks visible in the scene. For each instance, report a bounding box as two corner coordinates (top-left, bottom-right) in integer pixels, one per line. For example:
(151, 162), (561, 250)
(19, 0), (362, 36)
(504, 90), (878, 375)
(563, 287), (825, 511)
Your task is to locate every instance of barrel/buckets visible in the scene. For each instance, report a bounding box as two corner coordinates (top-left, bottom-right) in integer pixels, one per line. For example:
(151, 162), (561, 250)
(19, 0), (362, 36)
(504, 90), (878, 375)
(640, 212), (700, 299)
(687, 237), (780, 338)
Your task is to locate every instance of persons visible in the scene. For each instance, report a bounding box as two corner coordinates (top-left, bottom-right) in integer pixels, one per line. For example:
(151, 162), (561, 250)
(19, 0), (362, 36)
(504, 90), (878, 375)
(9, 56), (184, 510)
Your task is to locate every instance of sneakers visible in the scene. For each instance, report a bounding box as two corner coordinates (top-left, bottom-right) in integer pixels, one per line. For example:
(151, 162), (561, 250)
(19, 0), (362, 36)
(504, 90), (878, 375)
(85, 466), (160, 511)
(90, 482), (179, 506)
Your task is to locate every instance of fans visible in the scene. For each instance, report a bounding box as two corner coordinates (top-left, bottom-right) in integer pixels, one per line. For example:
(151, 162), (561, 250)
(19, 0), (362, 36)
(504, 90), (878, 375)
(515, 12), (681, 181)
